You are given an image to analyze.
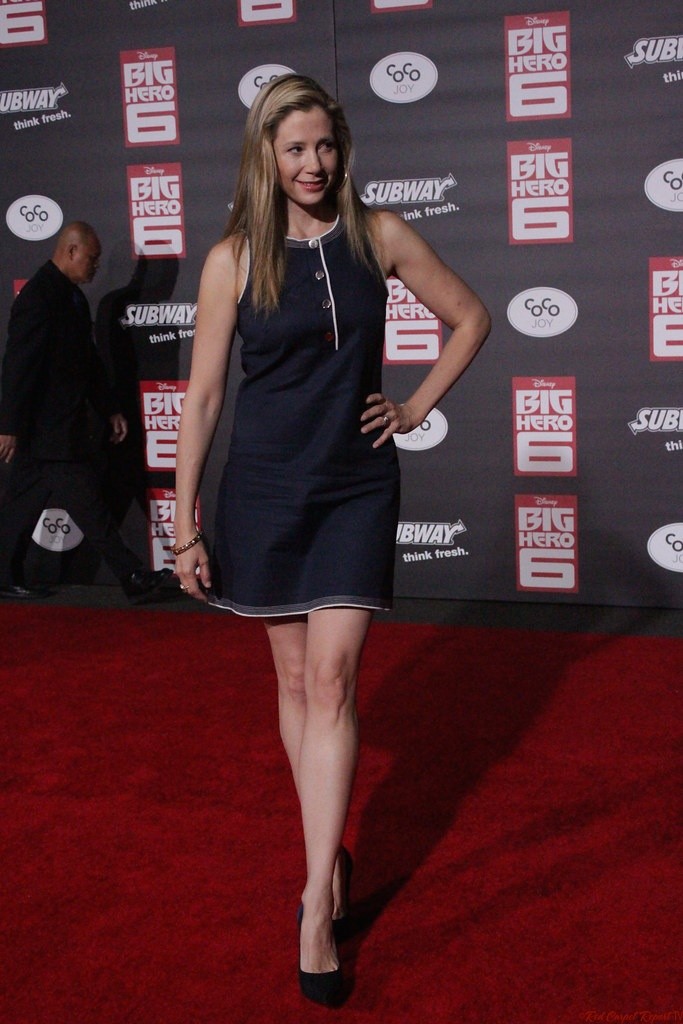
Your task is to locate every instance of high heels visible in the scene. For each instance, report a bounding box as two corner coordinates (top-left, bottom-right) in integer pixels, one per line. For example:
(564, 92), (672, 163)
(296, 846), (353, 1009)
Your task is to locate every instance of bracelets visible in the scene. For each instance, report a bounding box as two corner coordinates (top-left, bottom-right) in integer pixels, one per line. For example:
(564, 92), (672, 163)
(170, 530), (202, 555)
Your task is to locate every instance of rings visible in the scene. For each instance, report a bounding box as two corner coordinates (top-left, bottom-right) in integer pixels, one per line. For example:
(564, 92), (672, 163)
(381, 415), (390, 427)
(179, 585), (189, 592)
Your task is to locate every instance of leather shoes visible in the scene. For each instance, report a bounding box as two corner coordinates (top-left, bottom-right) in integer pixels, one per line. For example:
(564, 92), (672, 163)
(0, 583), (50, 599)
(119, 568), (173, 605)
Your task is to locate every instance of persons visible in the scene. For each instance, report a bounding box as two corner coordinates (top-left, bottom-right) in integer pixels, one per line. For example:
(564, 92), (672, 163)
(171, 75), (491, 1009)
(1, 221), (172, 604)
